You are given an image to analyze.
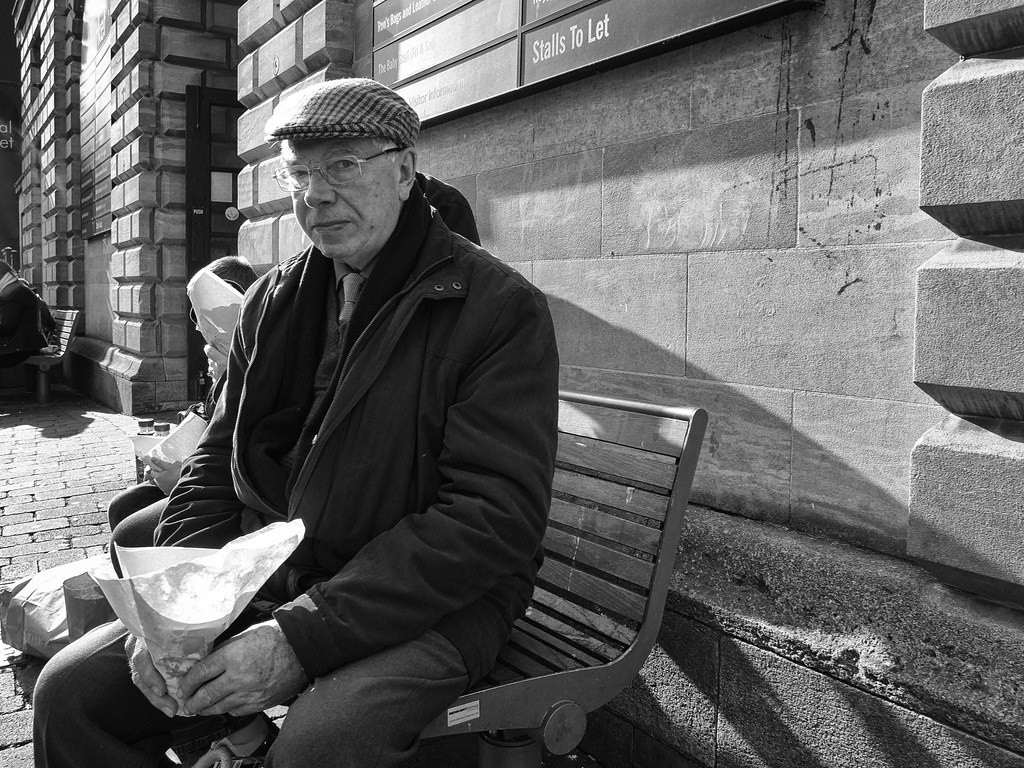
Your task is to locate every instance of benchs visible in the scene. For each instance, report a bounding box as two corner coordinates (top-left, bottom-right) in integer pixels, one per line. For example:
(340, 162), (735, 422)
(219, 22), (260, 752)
(421, 390), (709, 768)
(22, 310), (82, 407)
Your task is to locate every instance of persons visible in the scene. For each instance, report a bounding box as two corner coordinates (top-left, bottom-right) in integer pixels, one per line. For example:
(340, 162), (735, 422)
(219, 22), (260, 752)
(33, 78), (560, 768)
(0, 259), (45, 405)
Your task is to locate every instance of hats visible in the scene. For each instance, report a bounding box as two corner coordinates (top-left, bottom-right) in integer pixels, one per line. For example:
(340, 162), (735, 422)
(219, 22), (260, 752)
(270, 78), (421, 149)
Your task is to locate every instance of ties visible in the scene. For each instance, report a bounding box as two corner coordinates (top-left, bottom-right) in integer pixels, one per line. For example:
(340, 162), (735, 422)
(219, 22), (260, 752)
(339, 273), (365, 327)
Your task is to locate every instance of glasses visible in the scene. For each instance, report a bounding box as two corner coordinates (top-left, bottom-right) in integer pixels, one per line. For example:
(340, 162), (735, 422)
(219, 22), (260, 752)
(273, 148), (402, 192)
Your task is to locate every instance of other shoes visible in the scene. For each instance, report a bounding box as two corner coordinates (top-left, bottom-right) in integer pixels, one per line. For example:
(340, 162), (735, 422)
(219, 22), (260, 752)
(210, 712), (280, 767)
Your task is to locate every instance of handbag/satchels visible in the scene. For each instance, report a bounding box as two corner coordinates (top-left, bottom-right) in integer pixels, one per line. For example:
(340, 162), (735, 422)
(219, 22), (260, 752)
(0, 554), (124, 659)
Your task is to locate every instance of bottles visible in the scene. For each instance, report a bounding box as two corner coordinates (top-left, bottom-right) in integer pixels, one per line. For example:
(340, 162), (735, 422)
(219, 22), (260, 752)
(136, 419), (156, 487)
(152, 422), (170, 457)
